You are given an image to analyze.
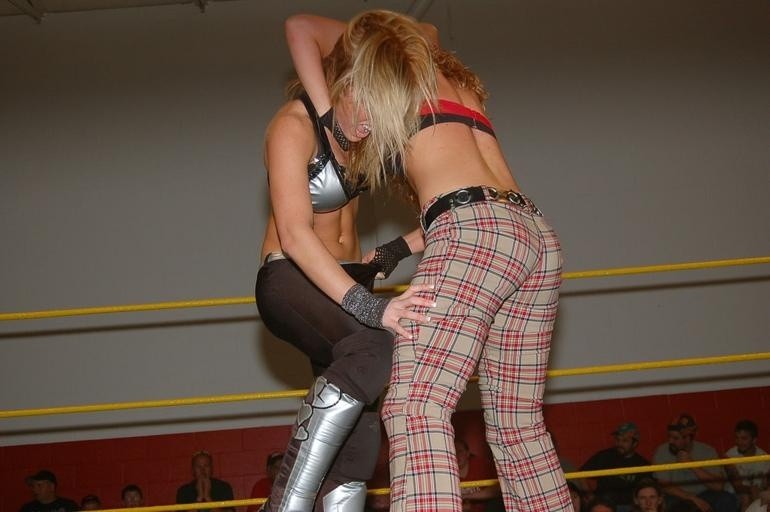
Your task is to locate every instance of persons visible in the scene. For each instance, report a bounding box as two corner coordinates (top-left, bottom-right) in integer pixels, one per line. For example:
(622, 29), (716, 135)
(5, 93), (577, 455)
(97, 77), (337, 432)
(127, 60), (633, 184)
(252, 8), (441, 512)
(20, 470), (78, 509)
(81, 497), (103, 510)
(252, 452), (285, 508)
(465, 431), (601, 509)
(650, 414), (738, 509)
(122, 485), (143, 508)
(177, 452), (234, 508)
(746, 472), (769, 511)
(454, 440), (484, 508)
(572, 423), (653, 509)
(721, 419), (768, 508)
(284, 12), (581, 511)
(632, 478), (663, 510)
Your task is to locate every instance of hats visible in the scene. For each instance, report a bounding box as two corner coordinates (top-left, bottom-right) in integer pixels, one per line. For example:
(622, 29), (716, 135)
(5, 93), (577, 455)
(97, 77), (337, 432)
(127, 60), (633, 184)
(610, 421), (640, 441)
(668, 411), (697, 435)
(24, 469), (59, 487)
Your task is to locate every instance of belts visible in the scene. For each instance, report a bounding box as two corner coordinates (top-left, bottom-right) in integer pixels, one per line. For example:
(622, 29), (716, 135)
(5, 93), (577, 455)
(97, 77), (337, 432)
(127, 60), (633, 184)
(425, 185), (544, 231)
